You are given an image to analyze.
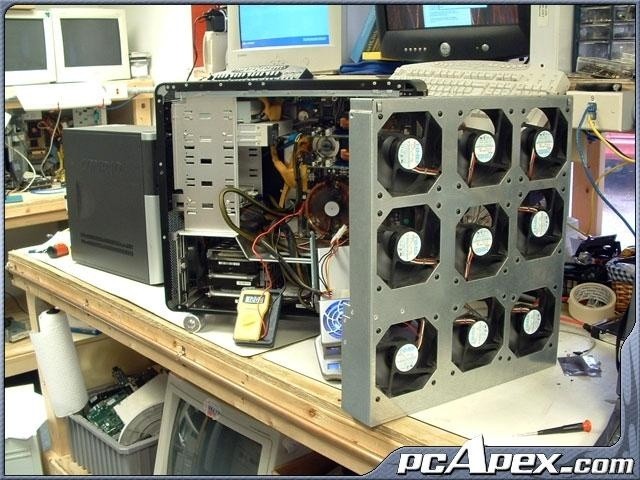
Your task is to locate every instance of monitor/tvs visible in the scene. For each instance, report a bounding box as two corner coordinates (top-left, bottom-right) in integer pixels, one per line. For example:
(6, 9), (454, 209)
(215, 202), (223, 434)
(223, 3), (375, 75)
(152, 371), (314, 476)
(4, 8), (132, 87)
(375, 3), (525, 64)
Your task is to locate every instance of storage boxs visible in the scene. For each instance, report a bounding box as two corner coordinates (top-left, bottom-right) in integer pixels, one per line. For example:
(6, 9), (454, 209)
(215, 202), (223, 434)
(66, 380), (159, 476)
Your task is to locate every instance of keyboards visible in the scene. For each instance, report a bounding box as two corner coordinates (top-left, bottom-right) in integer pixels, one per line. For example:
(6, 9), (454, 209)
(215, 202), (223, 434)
(201, 64), (314, 81)
(388, 59), (571, 96)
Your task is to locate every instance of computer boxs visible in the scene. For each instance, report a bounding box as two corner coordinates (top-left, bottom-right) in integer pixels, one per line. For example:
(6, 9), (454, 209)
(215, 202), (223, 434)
(61, 125), (165, 286)
(155, 78), (429, 318)
(3, 99), (107, 194)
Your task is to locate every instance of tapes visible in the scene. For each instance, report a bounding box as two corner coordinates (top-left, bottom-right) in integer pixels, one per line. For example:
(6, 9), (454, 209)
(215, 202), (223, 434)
(568, 282), (617, 326)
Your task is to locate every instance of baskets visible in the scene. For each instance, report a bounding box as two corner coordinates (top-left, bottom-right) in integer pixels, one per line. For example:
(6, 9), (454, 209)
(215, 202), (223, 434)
(606, 255), (635, 313)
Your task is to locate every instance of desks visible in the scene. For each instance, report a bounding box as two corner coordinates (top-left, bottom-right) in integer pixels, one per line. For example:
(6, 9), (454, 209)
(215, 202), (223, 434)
(6, 185), (74, 380)
(4, 245), (620, 475)
